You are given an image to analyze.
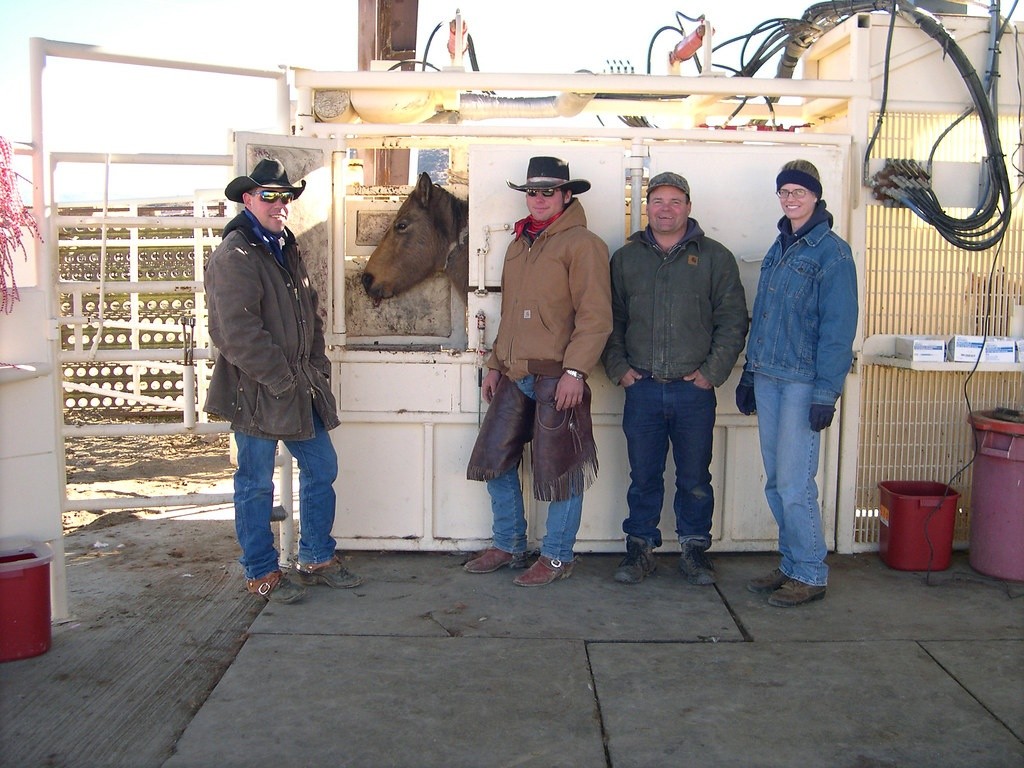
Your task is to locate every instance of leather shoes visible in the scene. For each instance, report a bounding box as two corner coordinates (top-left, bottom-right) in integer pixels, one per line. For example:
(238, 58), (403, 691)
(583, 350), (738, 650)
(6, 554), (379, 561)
(512, 554), (574, 586)
(463, 546), (527, 573)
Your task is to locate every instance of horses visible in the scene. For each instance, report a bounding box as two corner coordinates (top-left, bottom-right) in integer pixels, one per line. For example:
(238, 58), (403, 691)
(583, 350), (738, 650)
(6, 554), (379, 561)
(361, 171), (468, 303)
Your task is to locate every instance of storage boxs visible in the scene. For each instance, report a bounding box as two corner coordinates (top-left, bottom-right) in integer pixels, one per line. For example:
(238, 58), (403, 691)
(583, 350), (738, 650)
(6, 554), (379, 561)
(949, 334), (1024, 364)
(895, 336), (944, 364)
(877, 480), (962, 571)
(0, 534), (56, 662)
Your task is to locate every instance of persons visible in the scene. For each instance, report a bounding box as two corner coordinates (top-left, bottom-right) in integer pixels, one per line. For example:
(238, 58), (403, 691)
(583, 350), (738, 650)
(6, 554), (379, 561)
(463, 156), (614, 588)
(201, 159), (364, 603)
(600, 172), (749, 585)
(735, 159), (859, 608)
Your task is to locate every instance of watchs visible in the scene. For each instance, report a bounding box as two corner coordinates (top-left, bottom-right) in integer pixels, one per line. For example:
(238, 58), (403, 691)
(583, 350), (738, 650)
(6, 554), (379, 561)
(566, 369), (583, 380)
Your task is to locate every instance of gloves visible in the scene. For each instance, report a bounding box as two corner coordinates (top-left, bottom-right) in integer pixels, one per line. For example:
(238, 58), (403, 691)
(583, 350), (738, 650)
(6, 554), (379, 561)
(736, 384), (757, 416)
(809, 405), (836, 432)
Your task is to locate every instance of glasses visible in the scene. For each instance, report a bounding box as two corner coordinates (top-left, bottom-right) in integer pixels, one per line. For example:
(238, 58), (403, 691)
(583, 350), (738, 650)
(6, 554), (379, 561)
(775, 189), (814, 199)
(247, 190), (295, 204)
(526, 188), (567, 197)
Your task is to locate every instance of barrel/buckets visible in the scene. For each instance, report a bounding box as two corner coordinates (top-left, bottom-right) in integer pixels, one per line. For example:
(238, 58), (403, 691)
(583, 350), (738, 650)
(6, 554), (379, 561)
(969, 410), (1024, 583)
(877, 481), (961, 570)
(0, 536), (52, 662)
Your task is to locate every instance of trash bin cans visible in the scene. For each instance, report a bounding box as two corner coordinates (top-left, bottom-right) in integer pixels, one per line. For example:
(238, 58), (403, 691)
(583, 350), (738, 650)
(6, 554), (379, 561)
(968, 408), (1024, 582)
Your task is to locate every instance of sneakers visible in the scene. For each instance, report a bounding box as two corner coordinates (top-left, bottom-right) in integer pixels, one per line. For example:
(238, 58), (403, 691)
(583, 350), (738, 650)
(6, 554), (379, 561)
(678, 538), (717, 585)
(296, 555), (364, 589)
(246, 569), (306, 605)
(768, 578), (826, 608)
(746, 568), (790, 593)
(614, 535), (657, 582)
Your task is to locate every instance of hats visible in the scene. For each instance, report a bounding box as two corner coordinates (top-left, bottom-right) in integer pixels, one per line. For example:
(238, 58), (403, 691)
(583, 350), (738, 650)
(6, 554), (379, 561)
(224, 158), (306, 204)
(646, 172), (690, 201)
(776, 160), (822, 200)
(506, 157), (591, 195)
